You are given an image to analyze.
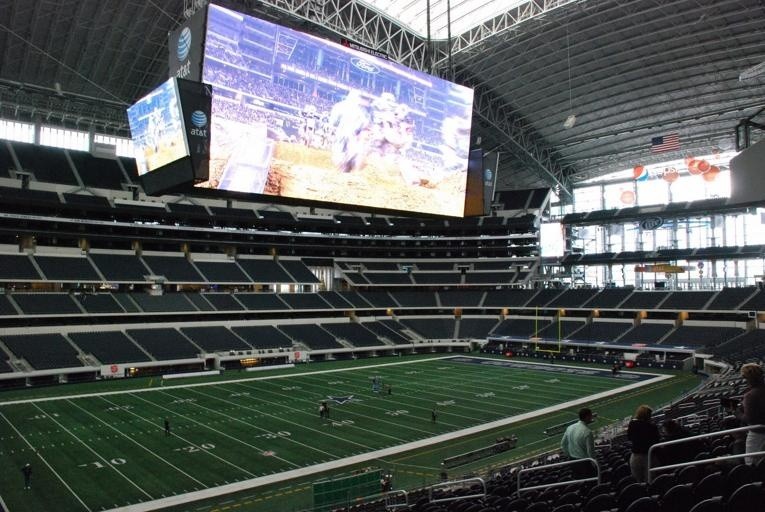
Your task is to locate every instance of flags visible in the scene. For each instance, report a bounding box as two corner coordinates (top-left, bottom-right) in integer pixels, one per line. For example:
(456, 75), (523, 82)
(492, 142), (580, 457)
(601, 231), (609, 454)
(650, 133), (682, 153)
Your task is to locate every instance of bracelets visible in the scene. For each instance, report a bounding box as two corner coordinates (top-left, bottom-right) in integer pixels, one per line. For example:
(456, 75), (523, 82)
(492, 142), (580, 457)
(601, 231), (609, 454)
(733, 410), (740, 415)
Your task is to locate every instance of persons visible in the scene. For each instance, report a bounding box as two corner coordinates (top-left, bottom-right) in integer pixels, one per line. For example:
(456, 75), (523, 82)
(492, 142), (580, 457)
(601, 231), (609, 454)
(323, 402), (330, 418)
(431, 407), (438, 423)
(319, 404), (324, 416)
(439, 459), (449, 480)
(206, 6), (476, 220)
(163, 419), (170, 435)
(560, 406), (596, 472)
(721, 363), (765, 467)
(379, 473), (392, 492)
(21, 463), (32, 489)
(626, 404), (663, 479)
(647, 419), (697, 476)
